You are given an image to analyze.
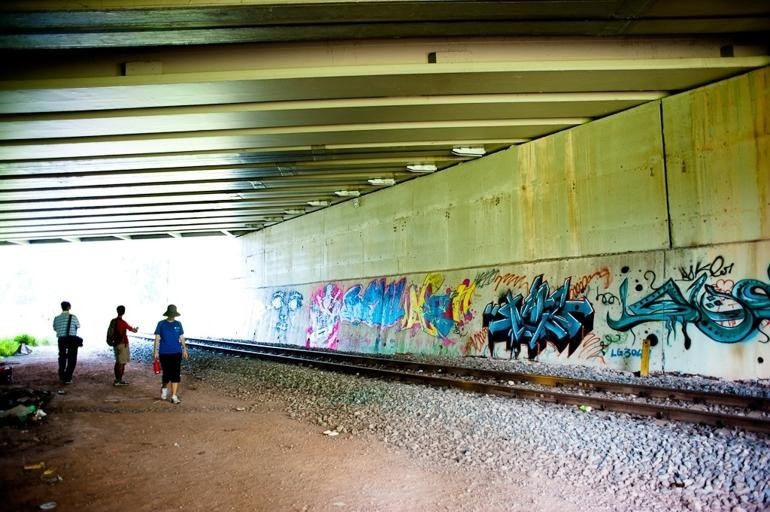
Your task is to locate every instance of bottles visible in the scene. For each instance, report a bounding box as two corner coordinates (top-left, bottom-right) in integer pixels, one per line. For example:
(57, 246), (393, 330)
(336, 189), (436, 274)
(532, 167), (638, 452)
(153, 359), (160, 375)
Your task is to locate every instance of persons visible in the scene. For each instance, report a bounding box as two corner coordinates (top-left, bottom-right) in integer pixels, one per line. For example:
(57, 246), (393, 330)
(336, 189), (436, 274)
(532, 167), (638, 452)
(153, 305), (188, 403)
(107, 306), (137, 384)
(53, 302), (80, 384)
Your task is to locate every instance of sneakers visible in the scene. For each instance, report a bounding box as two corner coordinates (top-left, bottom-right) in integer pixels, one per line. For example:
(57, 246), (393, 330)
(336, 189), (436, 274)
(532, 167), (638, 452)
(161, 386), (168, 400)
(171, 395), (180, 404)
(114, 379), (129, 386)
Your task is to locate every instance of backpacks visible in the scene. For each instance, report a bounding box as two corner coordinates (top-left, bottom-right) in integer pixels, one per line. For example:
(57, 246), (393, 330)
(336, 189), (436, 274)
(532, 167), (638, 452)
(106, 320), (122, 346)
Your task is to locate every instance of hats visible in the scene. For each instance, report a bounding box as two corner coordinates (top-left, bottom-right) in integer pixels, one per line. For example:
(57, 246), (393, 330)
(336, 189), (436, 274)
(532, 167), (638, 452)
(163, 304), (179, 316)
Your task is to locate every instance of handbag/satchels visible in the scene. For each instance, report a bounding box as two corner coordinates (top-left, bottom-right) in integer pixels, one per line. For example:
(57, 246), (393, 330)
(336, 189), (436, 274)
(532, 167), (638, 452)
(66, 335), (83, 348)
